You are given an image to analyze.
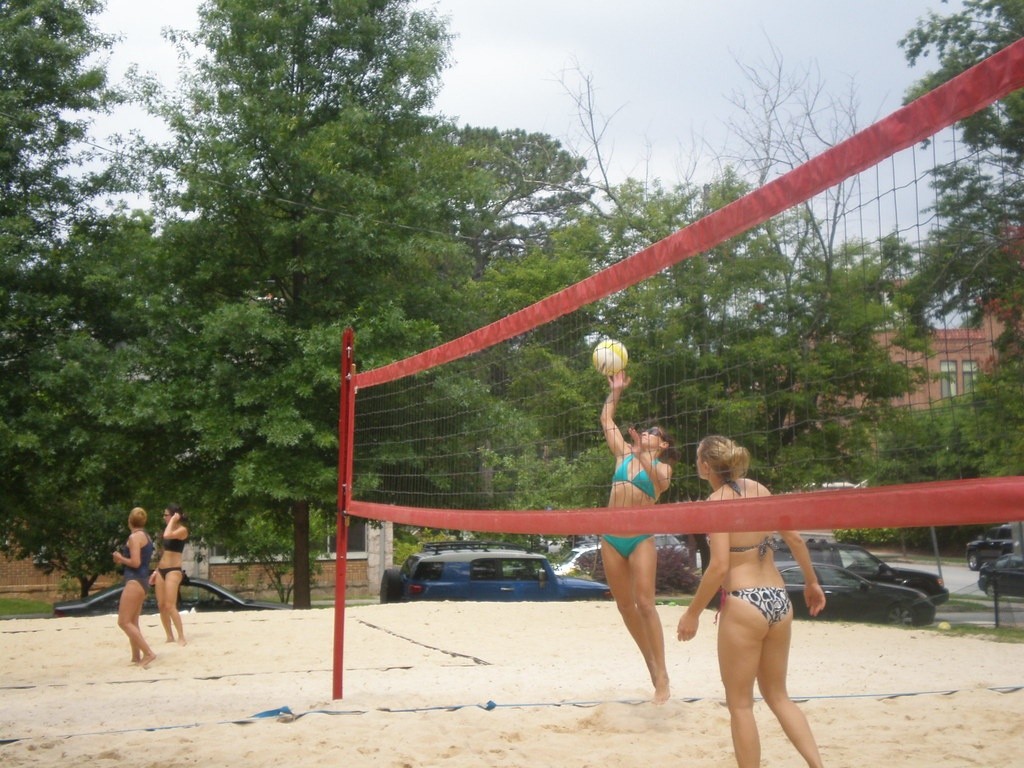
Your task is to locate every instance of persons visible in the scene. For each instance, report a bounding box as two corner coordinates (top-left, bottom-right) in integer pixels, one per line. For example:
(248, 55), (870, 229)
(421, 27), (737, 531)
(677, 433), (826, 768)
(112, 507), (156, 666)
(148, 504), (190, 647)
(600, 370), (674, 705)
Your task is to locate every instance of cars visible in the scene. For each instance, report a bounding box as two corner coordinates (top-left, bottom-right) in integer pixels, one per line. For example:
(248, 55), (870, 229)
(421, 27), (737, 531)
(54, 578), (293, 617)
(967, 524), (1024, 596)
(774, 544), (950, 626)
(553, 534), (701, 576)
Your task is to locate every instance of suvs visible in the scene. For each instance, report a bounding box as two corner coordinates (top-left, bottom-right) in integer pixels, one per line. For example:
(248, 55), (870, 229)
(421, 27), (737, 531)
(380, 542), (614, 604)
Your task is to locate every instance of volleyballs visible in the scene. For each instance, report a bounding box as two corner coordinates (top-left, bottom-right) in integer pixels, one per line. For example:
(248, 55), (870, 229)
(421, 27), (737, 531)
(938, 621), (951, 630)
(591, 339), (631, 375)
